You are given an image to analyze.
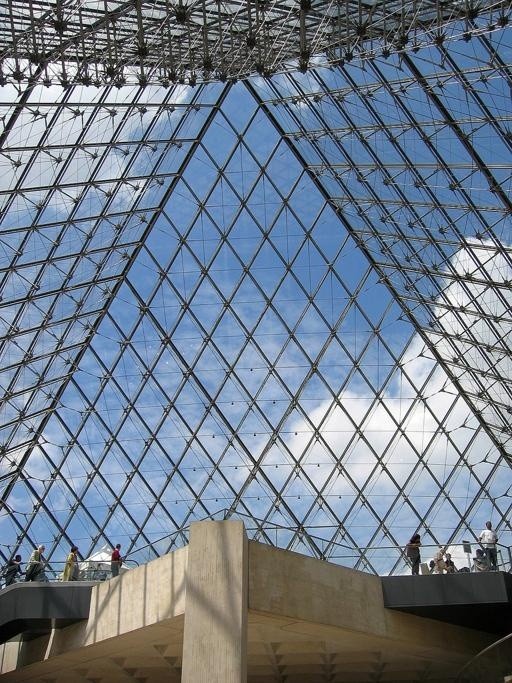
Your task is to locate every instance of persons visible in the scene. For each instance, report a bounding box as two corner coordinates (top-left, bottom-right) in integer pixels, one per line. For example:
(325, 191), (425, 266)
(406, 533), (422, 575)
(434, 547), (454, 573)
(67, 546), (79, 581)
(444, 552), (455, 573)
(111, 543), (126, 577)
(471, 549), (489, 571)
(5, 554), (26, 586)
(477, 520), (499, 571)
(24, 544), (46, 582)
